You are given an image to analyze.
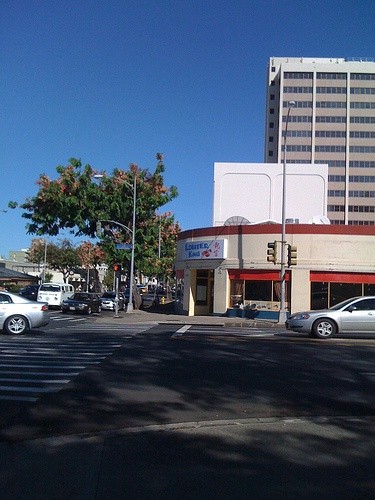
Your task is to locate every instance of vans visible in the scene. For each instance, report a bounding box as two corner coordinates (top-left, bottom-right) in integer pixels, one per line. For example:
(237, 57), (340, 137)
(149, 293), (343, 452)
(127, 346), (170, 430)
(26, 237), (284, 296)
(37, 283), (75, 310)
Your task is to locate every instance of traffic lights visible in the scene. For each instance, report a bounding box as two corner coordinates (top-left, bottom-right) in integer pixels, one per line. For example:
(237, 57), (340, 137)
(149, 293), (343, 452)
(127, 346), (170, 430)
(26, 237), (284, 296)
(286, 245), (298, 267)
(267, 240), (278, 262)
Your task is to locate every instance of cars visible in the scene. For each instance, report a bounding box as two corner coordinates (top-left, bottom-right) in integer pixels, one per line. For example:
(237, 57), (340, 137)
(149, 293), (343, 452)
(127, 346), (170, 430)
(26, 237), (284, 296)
(99, 291), (126, 311)
(124, 288), (144, 303)
(18, 284), (41, 300)
(60, 292), (102, 315)
(285, 295), (375, 338)
(0, 289), (51, 335)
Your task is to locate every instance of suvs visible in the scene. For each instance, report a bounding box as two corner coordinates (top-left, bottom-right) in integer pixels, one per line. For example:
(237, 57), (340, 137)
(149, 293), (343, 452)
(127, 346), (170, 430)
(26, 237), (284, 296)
(163, 284), (180, 296)
(126, 285), (147, 294)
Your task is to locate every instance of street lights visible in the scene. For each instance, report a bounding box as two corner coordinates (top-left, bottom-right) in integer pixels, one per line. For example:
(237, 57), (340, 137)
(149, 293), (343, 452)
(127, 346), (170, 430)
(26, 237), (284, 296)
(277, 101), (296, 324)
(114, 264), (122, 278)
(94, 174), (133, 313)
